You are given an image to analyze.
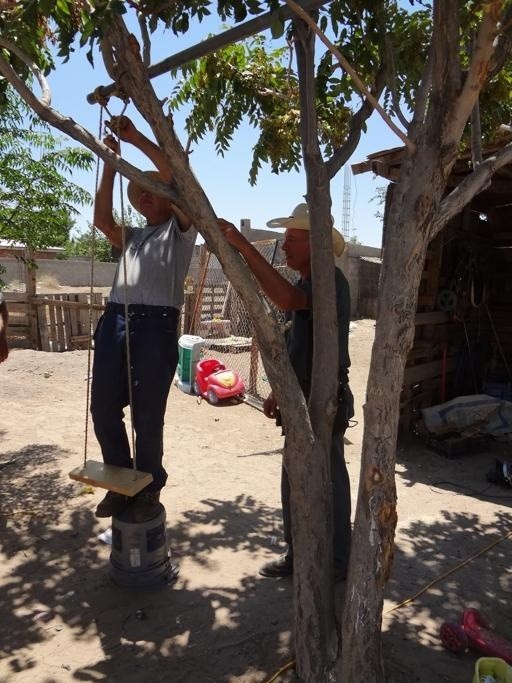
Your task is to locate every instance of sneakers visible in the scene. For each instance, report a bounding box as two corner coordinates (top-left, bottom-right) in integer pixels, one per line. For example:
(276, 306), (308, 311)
(92, 487), (132, 517)
(133, 484), (162, 523)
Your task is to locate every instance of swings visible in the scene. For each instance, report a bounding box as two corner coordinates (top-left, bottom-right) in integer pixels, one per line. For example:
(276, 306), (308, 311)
(68, 94), (153, 499)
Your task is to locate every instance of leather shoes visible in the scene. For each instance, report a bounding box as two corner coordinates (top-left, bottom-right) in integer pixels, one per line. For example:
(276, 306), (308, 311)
(258, 540), (356, 581)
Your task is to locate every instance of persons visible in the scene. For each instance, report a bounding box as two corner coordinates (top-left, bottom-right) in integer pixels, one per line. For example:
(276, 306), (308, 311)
(0, 287), (11, 367)
(89, 112), (199, 517)
(212, 202), (351, 584)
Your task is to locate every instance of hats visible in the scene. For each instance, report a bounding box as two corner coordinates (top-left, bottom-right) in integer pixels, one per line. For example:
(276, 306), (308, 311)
(264, 201), (349, 259)
(126, 169), (174, 218)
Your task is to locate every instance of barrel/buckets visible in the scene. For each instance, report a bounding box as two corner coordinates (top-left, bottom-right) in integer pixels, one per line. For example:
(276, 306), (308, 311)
(110, 500), (180, 591)
